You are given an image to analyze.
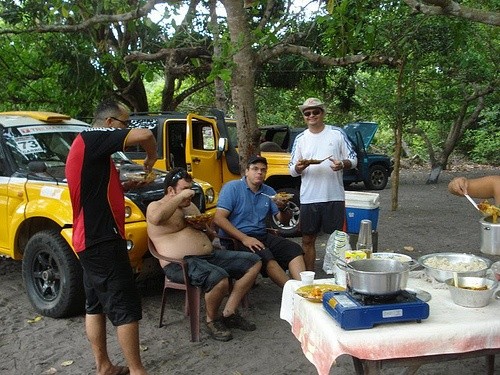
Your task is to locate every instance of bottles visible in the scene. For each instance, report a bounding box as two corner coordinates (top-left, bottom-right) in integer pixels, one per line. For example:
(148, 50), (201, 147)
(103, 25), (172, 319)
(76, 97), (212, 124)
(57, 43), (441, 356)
(356, 219), (373, 259)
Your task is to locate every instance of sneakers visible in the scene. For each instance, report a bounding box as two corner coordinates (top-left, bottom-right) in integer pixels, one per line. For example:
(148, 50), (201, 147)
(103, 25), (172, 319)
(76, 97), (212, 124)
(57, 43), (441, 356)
(221, 311), (256, 331)
(203, 315), (232, 341)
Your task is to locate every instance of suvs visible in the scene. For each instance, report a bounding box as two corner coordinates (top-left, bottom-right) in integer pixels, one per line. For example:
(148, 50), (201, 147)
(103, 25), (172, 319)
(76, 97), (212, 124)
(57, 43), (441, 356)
(117, 112), (310, 238)
(0, 110), (222, 318)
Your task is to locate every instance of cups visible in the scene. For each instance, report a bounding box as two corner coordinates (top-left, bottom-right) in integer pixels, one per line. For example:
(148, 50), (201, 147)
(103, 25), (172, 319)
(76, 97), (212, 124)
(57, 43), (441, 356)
(299, 271), (315, 286)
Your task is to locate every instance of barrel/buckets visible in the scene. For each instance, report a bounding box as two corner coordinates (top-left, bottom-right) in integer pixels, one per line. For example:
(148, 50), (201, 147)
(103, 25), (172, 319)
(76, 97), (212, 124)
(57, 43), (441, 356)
(479, 216), (500, 256)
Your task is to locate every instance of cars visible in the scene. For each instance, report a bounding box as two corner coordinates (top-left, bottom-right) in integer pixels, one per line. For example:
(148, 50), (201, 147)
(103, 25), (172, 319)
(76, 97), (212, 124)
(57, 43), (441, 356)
(271, 121), (393, 190)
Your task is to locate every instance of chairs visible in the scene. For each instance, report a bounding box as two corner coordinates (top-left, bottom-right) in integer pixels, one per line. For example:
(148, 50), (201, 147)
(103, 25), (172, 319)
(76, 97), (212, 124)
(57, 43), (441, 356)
(146, 227), (281, 343)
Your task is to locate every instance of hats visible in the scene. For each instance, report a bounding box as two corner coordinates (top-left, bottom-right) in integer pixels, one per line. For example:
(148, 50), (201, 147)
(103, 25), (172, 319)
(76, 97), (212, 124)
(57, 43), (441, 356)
(247, 154), (267, 165)
(298, 98), (327, 118)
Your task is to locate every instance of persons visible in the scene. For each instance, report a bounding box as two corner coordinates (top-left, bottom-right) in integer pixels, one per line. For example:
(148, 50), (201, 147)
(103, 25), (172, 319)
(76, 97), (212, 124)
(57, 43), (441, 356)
(146, 167), (262, 341)
(213, 155), (305, 289)
(448, 175), (500, 208)
(288, 98), (357, 271)
(65, 94), (158, 375)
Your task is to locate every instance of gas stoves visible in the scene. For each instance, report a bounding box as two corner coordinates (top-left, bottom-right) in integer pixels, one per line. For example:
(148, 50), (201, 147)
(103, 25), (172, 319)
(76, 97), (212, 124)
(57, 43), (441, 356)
(321, 290), (430, 331)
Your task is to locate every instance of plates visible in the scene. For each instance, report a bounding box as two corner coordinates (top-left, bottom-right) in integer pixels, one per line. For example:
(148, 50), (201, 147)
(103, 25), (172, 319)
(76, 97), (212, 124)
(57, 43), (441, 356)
(269, 194), (295, 199)
(299, 160), (324, 164)
(407, 288), (431, 302)
(123, 173), (156, 180)
(185, 215), (214, 220)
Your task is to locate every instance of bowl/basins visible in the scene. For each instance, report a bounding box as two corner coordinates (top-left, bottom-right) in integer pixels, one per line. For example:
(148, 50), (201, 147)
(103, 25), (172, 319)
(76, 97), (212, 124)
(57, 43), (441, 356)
(418, 252), (492, 283)
(372, 253), (412, 263)
(445, 277), (498, 308)
(295, 285), (346, 303)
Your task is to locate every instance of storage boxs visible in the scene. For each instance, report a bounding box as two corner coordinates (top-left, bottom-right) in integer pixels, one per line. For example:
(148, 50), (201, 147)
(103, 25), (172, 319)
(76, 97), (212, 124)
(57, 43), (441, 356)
(343, 190), (380, 233)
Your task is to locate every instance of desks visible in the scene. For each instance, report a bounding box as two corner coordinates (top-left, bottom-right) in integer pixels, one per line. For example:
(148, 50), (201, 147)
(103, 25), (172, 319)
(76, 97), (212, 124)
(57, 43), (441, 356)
(279, 278), (500, 375)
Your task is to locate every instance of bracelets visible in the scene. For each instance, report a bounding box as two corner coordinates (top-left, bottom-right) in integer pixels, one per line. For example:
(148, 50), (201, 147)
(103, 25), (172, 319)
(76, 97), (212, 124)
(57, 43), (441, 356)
(340, 161), (344, 168)
(203, 226), (209, 232)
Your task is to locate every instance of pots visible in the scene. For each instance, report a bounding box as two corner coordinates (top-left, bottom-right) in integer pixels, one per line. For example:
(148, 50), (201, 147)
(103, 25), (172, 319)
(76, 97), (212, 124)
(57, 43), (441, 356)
(335, 256), (420, 297)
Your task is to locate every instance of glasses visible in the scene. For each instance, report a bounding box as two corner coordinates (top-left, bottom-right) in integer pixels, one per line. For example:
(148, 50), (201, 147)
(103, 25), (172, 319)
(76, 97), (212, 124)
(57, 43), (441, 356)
(105, 116), (130, 128)
(171, 171), (185, 184)
(304, 110), (322, 116)
